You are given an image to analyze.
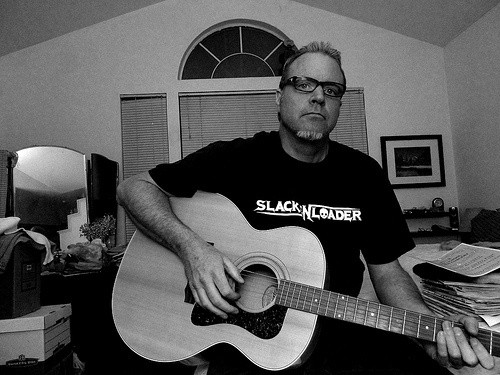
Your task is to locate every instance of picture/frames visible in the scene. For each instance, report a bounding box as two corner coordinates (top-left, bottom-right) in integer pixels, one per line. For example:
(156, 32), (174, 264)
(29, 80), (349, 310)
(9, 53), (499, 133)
(380, 134), (446, 189)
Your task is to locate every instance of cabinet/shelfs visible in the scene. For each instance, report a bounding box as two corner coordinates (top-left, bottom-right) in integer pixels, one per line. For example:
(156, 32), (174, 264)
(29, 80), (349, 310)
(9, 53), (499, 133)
(40, 269), (128, 375)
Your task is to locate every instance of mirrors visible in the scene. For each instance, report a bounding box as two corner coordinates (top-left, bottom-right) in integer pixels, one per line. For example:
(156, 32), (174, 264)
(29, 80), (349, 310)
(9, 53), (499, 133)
(12, 145), (89, 250)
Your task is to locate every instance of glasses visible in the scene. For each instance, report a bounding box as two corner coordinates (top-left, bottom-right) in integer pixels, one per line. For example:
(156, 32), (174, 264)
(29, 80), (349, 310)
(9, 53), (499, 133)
(280, 75), (346, 98)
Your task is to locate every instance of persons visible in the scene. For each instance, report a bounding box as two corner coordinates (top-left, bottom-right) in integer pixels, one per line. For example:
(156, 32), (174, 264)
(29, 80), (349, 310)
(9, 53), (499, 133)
(116, 42), (494, 375)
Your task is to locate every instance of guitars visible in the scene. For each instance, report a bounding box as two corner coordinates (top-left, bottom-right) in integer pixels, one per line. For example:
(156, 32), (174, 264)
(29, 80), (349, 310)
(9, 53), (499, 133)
(111, 190), (500, 371)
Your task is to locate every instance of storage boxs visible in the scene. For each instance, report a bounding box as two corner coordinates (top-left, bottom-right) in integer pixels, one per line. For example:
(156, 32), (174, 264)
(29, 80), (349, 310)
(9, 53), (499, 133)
(0, 304), (73, 364)
(0, 244), (40, 319)
